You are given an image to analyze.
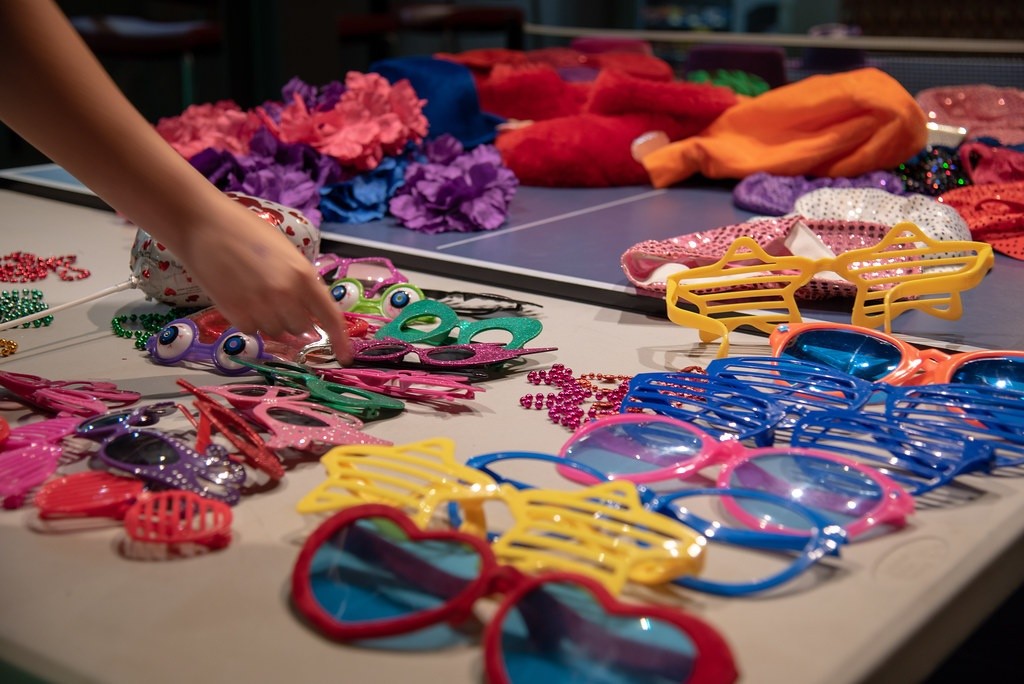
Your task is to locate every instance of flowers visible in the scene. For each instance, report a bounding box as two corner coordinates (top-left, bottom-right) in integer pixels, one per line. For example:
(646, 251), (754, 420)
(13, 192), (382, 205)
(155, 70), (518, 235)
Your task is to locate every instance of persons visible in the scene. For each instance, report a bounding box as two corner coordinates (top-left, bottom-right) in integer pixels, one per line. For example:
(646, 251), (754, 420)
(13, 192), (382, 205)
(0, 0), (346, 360)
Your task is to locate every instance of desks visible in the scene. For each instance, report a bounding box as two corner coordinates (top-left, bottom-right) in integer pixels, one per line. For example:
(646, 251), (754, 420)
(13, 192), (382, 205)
(0, 191), (1024, 684)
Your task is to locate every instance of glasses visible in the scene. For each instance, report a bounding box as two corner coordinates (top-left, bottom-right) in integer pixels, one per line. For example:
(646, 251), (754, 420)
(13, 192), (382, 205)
(701, 356), (1022, 469)
(555, 413), (916, 537)
(295, 435), (709, 600)
(665, 222), (993, 359)
(768, 321), (1023, 441)
(446, 452), (846, 596)
(618, 372), (993, 498)
(290, 503), (741, 684)
(0, 254), (558, 552)
